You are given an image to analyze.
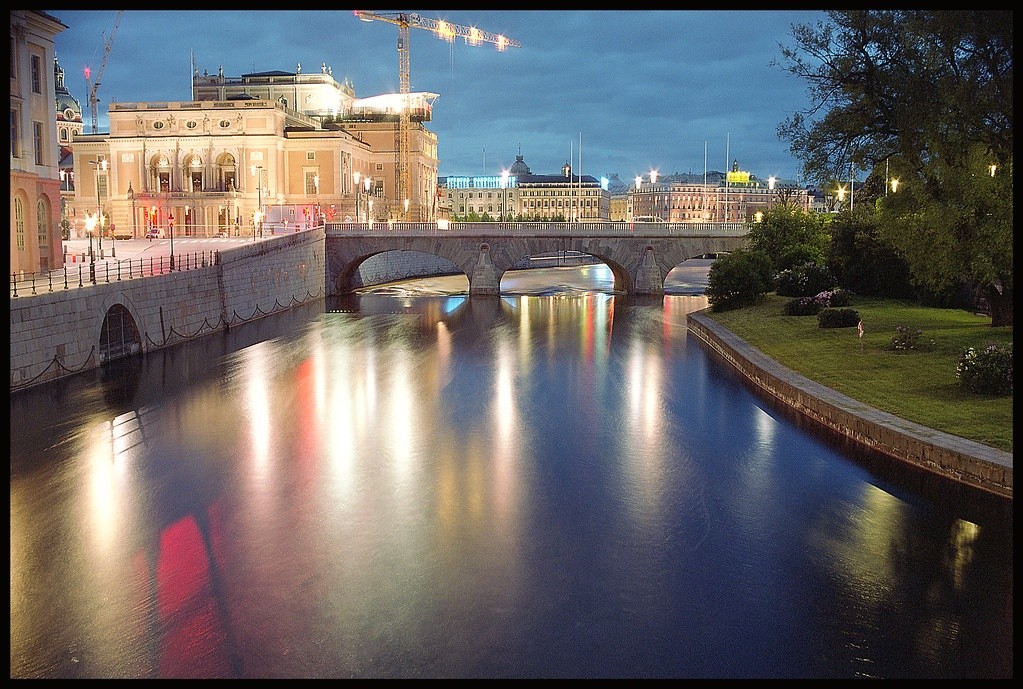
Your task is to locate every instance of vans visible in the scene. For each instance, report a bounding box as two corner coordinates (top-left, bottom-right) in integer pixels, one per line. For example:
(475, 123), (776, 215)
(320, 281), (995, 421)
(635, 215), (665, 222)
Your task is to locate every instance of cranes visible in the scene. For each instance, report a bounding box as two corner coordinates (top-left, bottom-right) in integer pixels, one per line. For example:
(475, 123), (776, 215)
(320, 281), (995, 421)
(354, 9), (523, 222)
(82, 10), (124, 135)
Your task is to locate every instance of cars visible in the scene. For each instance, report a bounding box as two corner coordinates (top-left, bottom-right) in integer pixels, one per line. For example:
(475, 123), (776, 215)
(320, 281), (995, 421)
(146, 228), (165, 239)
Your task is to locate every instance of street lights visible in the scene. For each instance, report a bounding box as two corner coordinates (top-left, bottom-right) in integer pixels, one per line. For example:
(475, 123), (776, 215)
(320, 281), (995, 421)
(251, 210), (259, 241)
(353, 170), (361, 222)
(251, 166), (266, 239)
(167, 213), (175, 270)
(365, 178), (370, 222)
(89, 160), (106, 249)
(86, 219), (96, 281)
